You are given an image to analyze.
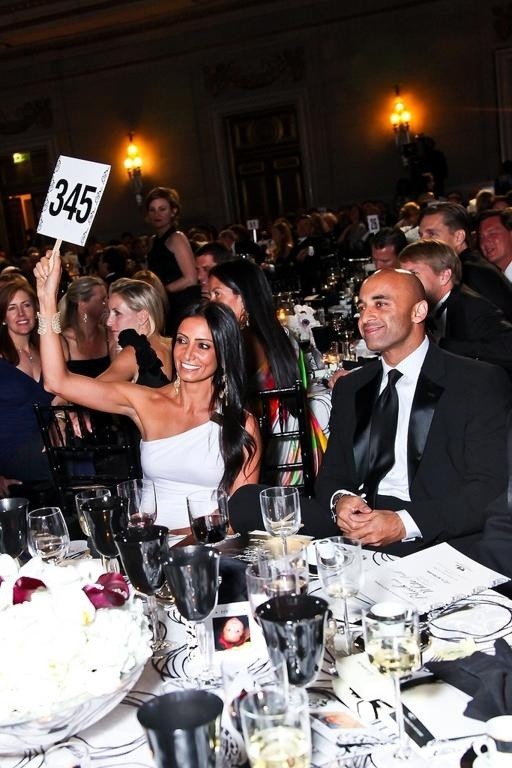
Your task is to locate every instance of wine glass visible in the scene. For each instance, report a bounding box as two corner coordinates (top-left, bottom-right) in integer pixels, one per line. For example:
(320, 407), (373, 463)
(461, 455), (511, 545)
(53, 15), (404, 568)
(328, 314), (355, 364)
(312, 533), (363, 667)
(186, 487), (231, 551)
(160, 541), (226, 678)
(79, 494), (129, 580)
(74, 485), (112, 580)
(257, 483), (301, 555)
(270, 289), (302, 322)
(257, 539), (309, 598)
(242, 555), (300, 612)
(324, 263), (365, 301)
(113, 523), (178, 656)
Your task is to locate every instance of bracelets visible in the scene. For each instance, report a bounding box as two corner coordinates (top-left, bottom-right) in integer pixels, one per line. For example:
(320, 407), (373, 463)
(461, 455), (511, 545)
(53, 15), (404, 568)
(329, 494), (352, 524)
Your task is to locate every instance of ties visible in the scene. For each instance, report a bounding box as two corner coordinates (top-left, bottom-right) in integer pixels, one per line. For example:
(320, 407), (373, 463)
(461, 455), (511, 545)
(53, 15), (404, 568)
(361, 369), (404, 507)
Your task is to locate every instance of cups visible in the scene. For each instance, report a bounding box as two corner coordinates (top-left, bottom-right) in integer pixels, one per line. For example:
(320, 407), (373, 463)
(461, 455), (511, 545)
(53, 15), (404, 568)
(114, 477), (157, 530)
(25, 506), (71, 570)
(252, 592), (329, 690)
(135, 686), (226, 768)
(0, 496), (31, 571)
(235, 681), (314, 768)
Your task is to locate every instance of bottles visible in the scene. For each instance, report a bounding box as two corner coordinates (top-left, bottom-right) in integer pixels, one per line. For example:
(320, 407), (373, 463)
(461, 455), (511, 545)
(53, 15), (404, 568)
(362, 597), (423, 675)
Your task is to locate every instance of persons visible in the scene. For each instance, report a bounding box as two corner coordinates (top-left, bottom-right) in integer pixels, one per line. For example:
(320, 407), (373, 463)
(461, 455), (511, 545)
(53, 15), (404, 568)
(225, 265), (510, 559)
(1, 173), (511, 504)
(214, 614), (251, 651)
(30, 248), (263, 546)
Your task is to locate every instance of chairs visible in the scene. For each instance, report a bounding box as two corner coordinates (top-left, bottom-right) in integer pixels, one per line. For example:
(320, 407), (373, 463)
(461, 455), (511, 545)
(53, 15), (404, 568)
(246, 378), (314, 499)
(35, 395), (147, 537)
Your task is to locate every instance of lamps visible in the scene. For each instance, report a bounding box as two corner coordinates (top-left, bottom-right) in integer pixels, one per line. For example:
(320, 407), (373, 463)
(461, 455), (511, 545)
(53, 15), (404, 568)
(116, 129), (154, 211)
(386, 87), (414, 164)
